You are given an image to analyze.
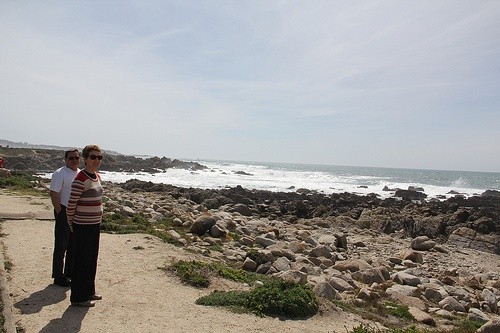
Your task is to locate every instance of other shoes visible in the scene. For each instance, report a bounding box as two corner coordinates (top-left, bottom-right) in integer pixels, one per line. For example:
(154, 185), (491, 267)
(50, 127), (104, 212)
(90, 294), (102, 300)
(53, 274), (71, 286)
(71, 300), (95, 306)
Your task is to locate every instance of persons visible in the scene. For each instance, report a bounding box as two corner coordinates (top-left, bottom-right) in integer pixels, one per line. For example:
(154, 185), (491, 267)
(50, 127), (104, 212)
(66, 143), (104, 308)
(50, 146), (82, 288)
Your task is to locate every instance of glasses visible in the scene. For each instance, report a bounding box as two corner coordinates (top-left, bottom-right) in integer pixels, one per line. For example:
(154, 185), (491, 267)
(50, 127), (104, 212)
(67, 156), (79, 160)
(87, 155), (103, 160)
